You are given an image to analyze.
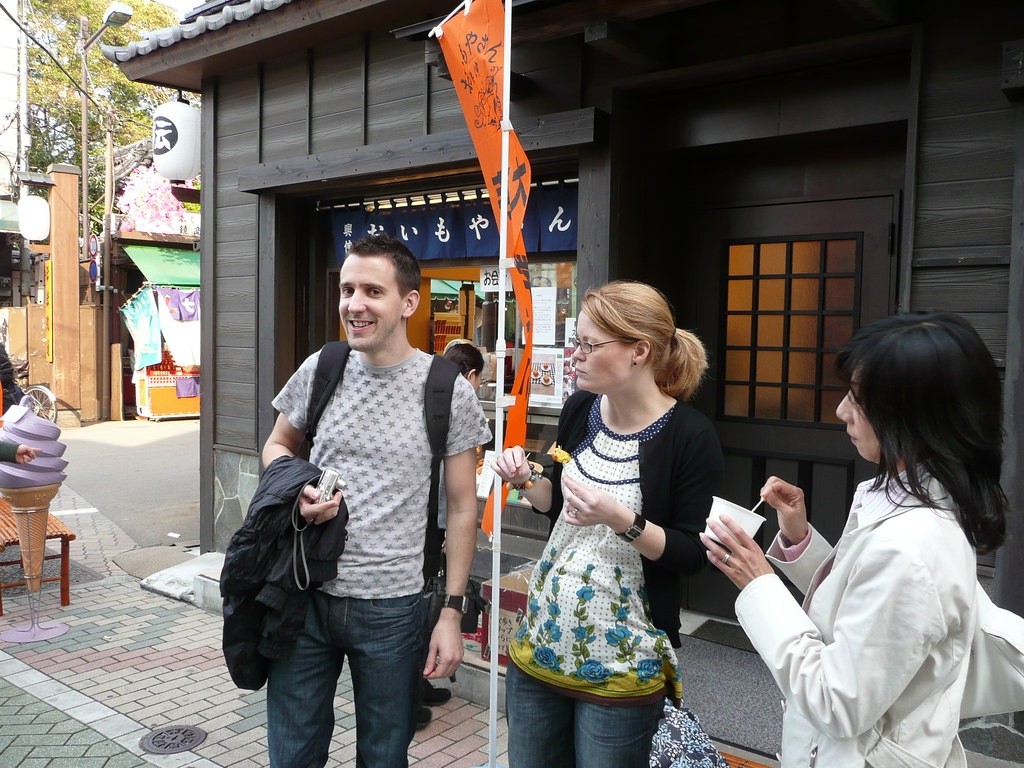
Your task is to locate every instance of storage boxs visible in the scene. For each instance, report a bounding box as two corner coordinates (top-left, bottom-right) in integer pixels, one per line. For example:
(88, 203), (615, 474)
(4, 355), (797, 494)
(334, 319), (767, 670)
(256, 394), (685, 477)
(481, 564), (531, 663)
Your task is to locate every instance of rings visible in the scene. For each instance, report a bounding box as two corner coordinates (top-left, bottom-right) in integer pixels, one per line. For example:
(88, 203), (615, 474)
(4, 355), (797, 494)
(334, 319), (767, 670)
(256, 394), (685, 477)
(569, 508), (578, 519)
(721, 551), (731, 564)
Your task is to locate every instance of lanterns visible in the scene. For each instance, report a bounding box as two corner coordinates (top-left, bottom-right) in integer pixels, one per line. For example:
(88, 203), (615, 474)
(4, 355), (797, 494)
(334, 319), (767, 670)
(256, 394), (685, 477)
(18, 194), (51, 244)
(152, 98), (202, 184)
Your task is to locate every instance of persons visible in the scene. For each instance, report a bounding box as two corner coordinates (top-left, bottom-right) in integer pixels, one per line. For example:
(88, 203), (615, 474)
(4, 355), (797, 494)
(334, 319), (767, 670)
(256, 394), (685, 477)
(263, 233), (493, 768)
(699, 311), (1009, 768)
(0, 441), (43, 465)
(0, 342), (25, 414)
(490, 283), (729, 768)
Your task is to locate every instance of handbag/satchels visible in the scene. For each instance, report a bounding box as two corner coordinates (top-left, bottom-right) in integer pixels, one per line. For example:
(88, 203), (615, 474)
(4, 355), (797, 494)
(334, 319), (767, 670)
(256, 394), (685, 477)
(650, 698), (731, 768)
(961, 585), (1023, 719)
(422, 564), (480, 633)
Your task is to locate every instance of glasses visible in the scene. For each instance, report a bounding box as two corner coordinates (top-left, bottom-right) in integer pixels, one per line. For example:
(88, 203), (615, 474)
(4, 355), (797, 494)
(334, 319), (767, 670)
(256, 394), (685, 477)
(571, 337), (624, 354)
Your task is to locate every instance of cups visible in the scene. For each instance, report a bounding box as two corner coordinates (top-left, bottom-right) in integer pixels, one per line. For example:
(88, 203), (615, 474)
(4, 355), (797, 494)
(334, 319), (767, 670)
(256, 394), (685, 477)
(705, 496), (767, 545)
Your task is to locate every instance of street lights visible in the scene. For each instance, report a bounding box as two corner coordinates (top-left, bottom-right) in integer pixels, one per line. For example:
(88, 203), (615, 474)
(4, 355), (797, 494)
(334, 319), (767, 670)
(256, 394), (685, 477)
(74, 0), (132, 432)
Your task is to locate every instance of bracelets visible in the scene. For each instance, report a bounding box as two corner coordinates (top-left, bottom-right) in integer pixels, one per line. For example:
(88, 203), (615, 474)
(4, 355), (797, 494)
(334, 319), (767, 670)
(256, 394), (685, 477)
(506, 459), (543, 494)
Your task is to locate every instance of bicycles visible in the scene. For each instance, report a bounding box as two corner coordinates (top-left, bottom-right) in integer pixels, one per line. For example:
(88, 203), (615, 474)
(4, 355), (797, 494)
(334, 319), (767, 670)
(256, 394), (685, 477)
(18, 371), (58, 423)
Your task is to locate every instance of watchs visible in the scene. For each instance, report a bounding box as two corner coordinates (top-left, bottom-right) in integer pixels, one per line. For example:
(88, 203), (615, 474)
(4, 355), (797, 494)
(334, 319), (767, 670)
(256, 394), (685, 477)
(440, 594), (468, 614)
(615, 512), (646, 542)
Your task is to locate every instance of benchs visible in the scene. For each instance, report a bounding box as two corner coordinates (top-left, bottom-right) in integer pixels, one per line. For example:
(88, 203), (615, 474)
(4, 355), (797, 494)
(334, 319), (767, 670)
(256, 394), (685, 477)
(0, 498), (76, 618)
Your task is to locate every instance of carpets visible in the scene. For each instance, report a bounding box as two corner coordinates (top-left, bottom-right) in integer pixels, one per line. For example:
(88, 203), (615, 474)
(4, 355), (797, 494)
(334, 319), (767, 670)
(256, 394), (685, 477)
(670, 634), (784, 760)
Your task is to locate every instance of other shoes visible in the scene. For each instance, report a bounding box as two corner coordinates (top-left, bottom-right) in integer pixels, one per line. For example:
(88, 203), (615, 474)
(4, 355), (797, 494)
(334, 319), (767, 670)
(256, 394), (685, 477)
(417, 672), (451, 707)
(414, 707), (432, 729)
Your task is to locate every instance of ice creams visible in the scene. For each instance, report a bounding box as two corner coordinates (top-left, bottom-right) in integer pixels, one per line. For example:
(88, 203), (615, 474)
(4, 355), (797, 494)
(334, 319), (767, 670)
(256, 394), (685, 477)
(0, 395), (69, 594)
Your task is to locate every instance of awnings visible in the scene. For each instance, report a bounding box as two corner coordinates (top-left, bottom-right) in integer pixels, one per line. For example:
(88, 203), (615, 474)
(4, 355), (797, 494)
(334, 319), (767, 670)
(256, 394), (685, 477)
(0, 200), (21, 234)
(118, 244), (201, 287)
(430, 278), (516, 305)
